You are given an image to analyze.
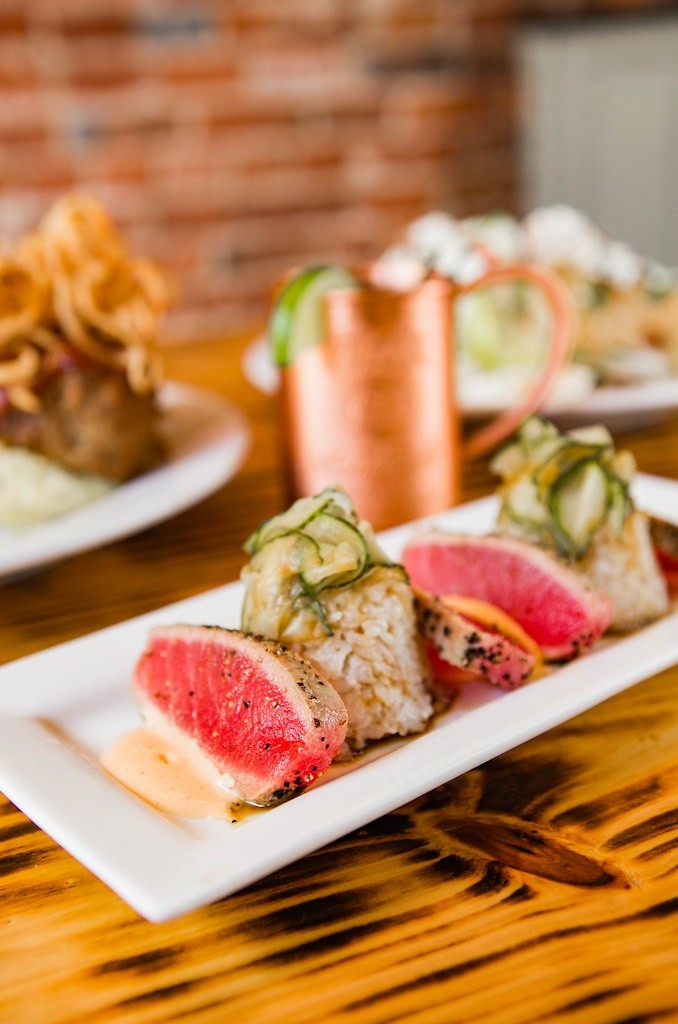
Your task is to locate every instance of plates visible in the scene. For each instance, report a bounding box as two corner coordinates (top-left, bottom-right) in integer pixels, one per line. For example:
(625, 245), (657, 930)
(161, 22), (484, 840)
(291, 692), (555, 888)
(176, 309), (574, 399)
(0, 473), (678, 923)
(245, 335), (678, 441)
(0, 375), (250, 577)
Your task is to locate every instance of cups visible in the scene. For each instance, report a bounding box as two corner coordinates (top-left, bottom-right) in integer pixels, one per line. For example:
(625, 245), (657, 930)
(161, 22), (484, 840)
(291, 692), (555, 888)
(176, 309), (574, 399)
(276, 267), (574, 534)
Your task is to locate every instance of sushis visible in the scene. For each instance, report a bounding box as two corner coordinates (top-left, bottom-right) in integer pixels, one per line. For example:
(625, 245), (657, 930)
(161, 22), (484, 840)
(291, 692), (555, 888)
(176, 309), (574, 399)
(128, 415), (665, 809)
